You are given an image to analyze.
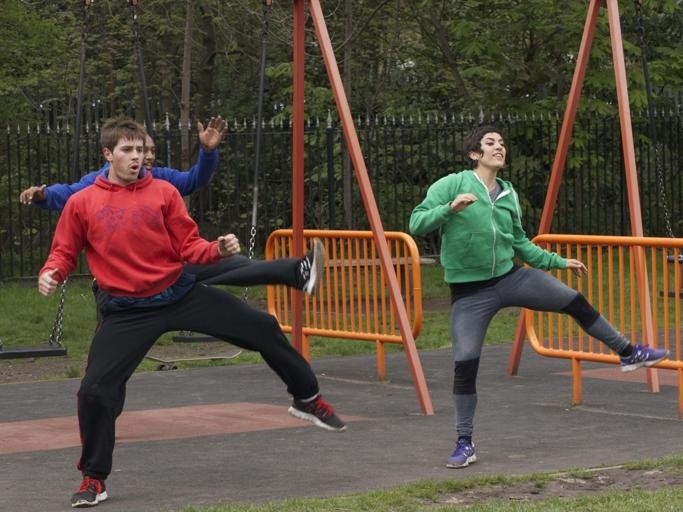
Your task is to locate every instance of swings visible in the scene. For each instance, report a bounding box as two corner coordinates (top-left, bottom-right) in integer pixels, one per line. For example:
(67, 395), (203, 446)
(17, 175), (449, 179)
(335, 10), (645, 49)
(131, 1), (273, 363)
(636, 1), (683, 263)
(0, 0), (92, 359)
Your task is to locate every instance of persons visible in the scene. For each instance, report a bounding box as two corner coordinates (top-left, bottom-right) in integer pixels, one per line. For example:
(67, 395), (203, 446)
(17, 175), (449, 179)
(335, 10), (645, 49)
(20, 116), (325, 295)
(409, 126), (669, 468)
(38, 117), (347, 508)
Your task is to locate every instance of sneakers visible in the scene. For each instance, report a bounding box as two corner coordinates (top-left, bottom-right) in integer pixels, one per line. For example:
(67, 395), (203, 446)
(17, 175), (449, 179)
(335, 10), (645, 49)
(444, 438), (479, 470)
(285, 394), (348, 434)
(618, 343), (670, 374)
(293, 236), (327, 298)
(68, 471), (109, 509)
